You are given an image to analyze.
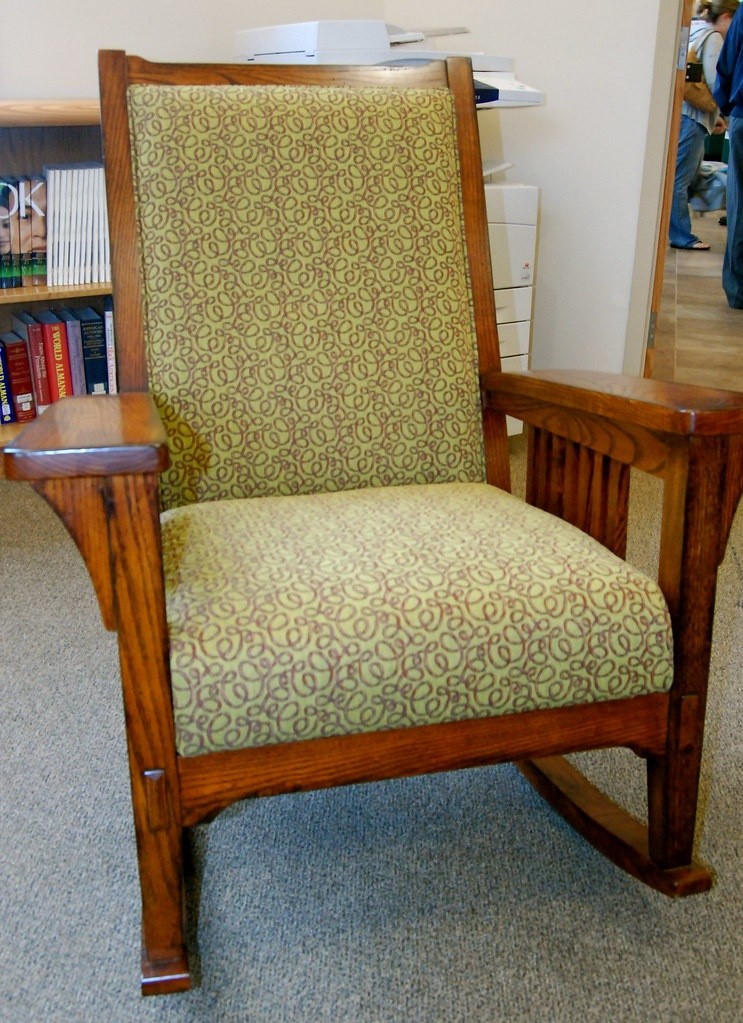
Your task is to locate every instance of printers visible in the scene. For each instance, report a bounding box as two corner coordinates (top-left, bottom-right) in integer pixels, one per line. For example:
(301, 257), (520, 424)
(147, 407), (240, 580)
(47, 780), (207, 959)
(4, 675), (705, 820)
(237, 20), (545, 439)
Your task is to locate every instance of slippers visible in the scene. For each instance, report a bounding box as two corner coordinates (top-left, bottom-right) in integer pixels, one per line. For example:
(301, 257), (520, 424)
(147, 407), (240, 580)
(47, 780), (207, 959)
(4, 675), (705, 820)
(670, 238), (710, 250)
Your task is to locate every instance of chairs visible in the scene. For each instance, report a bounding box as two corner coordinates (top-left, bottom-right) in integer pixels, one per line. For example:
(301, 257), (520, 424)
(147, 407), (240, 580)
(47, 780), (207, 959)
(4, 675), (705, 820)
(0, 50), (743, 996)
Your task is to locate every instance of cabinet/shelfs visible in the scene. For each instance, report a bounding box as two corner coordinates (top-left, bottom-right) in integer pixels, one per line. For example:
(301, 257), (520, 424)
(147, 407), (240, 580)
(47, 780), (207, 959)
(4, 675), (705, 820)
(478, 182), (539, 437)
(0, 99), (114, 454)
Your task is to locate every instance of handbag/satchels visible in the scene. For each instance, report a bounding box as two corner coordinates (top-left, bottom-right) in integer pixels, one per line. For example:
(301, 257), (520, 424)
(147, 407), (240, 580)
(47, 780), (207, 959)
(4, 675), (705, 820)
(682, 28), (717, 112)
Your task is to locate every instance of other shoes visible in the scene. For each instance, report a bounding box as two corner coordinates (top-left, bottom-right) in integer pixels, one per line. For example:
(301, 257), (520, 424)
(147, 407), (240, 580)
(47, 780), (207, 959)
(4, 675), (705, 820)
(718, 216), (727, 226)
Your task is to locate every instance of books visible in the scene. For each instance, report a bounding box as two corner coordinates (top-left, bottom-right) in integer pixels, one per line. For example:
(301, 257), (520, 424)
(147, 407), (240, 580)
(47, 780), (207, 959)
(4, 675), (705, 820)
(0, 160), (112, 289)
(0, 294), (118, 425)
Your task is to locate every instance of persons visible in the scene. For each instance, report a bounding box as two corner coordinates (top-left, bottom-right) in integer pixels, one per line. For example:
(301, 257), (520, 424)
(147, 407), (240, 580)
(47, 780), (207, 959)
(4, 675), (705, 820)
(692, 2), (713, 21)
(712, 1), (743, 310)
(0, 180), (47, 255)
(668, 0), (740, 251)
